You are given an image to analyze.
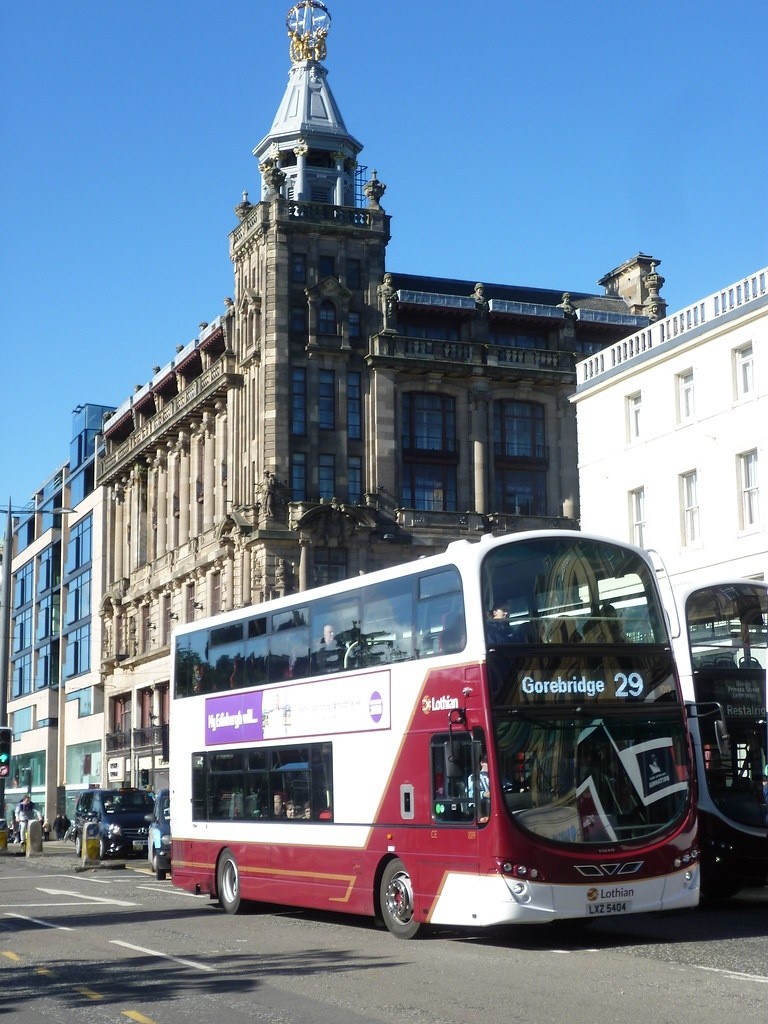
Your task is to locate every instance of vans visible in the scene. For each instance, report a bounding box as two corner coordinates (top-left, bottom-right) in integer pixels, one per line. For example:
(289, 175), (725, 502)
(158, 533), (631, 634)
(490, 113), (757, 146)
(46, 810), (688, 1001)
(70, 786), (155, 860)
(144, 788), (171, 881)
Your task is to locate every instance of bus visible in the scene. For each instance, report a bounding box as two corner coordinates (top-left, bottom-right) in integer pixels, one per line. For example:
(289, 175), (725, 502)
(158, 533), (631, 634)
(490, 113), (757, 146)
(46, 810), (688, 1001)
(168, 527), (733, 942)
(343, 573), (768, 906)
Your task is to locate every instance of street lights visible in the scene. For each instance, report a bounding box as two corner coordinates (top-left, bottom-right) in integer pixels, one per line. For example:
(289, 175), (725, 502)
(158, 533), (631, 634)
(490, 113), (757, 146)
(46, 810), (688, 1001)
(0, 496), (78, 833)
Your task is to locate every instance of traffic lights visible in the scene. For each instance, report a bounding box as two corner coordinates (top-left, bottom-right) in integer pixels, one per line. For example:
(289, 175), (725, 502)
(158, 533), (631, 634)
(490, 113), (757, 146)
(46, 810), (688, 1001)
(0, 727), (11, 779)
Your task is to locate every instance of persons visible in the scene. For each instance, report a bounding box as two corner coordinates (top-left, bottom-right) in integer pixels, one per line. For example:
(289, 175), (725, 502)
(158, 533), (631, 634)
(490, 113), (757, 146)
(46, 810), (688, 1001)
(192, 663), (207, 694)
(376, 273), (399, 331)
(53, 813), (71, 841)
(273, 791), (310, 820)
(583, 604), (631, 643)
(316, 624), (348, 674)
(762, 765), (768, 822)
(104, 796), (117, 813)
(259, 470), (275, 517)
(484, 600), (581, 642)
(102, 297), (234, 456)
(41, 817), (51, 841)
(467, 753), (490, 808)
(9, 795), (34, 845)
(470, 283), (490, 343)
